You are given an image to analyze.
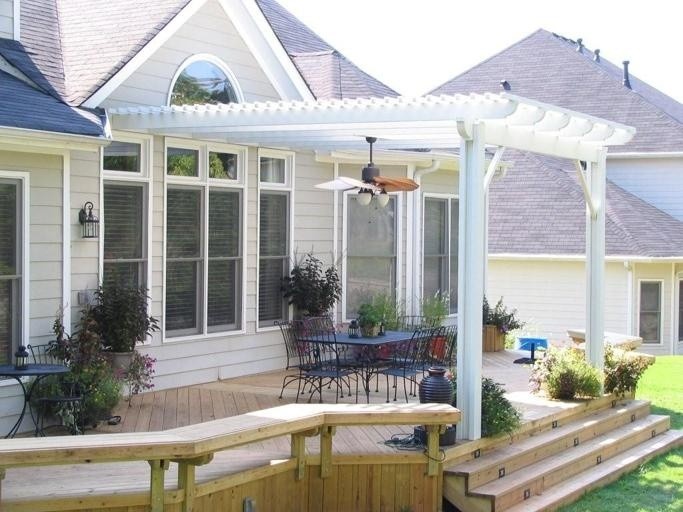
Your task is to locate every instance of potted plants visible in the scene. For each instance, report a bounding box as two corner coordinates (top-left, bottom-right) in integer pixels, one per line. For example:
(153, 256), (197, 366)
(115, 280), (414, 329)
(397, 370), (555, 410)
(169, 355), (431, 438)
(357, 303), (383, 339)
(366, 291), (405, 361)
(417, 289), (450, 363)
(26, 257), (161, 436)
(529, 343), (602, 400)
(279, 244), (343, 354)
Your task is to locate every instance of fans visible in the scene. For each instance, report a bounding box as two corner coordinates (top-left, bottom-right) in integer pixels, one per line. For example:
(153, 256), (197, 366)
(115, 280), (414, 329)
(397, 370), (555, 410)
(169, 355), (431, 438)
(314, 137), (419, 191)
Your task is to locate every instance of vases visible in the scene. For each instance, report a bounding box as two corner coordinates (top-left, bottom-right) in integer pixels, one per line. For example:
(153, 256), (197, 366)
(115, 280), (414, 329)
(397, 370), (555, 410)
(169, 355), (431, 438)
(547, 381), (576, 399)
(482, 325), (505, 352)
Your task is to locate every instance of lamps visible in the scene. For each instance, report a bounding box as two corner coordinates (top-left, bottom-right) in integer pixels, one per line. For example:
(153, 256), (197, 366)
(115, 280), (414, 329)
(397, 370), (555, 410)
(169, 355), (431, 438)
(357, 185), (389, 206)
(78, 201), (99, 239)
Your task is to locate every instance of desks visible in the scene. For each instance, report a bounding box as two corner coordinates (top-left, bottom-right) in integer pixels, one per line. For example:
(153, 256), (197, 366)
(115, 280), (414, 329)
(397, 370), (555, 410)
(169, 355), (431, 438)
(0, 363), (71, 437)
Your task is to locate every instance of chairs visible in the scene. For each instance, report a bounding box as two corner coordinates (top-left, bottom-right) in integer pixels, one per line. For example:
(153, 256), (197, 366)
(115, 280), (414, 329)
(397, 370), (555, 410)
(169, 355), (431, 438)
(274, 316), (457, 404)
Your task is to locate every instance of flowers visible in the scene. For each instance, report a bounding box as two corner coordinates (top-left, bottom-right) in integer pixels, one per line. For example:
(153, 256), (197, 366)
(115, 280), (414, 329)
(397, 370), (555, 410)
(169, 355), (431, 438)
(603, 341), (656, 398)
(483, 294), (526, 334)
(443, 367), (524, 446)
